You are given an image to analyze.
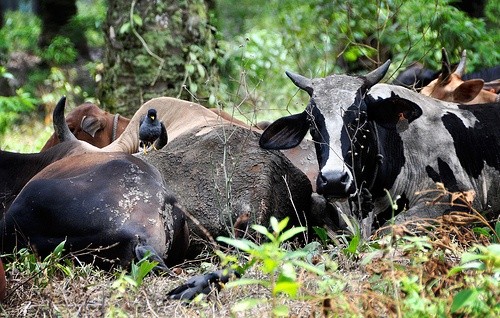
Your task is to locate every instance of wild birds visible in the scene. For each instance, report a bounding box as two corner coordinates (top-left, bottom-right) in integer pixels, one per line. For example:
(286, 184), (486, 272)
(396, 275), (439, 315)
(165, 272), (241, 307)
(138, 109), (168, 151)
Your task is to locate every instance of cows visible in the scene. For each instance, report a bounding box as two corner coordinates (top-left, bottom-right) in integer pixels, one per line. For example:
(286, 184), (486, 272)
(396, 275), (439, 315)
(0, 46), (500, 272)
